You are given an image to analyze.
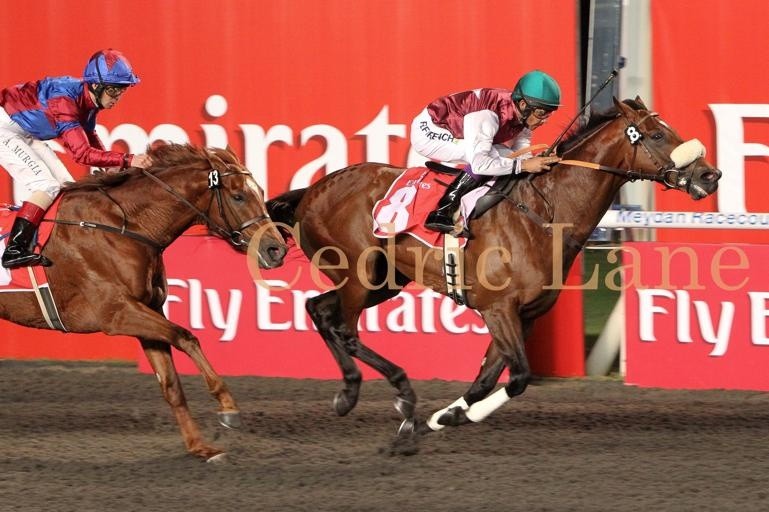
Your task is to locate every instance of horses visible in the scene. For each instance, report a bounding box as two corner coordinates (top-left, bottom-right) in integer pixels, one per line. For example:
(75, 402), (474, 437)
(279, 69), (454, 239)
(266, 92), (723, 442)
(0, 142), (290, 464)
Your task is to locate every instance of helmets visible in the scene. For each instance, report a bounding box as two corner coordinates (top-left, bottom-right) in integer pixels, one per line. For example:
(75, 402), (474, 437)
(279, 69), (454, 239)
(510, 69), (565, 110)
(80, 46), (141, 87)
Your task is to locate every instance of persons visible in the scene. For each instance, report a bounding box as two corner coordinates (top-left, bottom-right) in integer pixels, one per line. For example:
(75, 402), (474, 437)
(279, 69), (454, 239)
(0, 49), (154, 268)
(410, 70), (564, 238)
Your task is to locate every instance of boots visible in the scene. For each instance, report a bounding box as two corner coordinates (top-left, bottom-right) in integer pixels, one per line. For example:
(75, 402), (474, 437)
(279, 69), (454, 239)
(423, 170), (478, 238)
(1, 215), (51, 269)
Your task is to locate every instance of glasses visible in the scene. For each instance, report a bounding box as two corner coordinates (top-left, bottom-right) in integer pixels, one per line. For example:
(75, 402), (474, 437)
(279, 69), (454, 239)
(105, 87), (127, 96)
(529, 108), (551, 118)
